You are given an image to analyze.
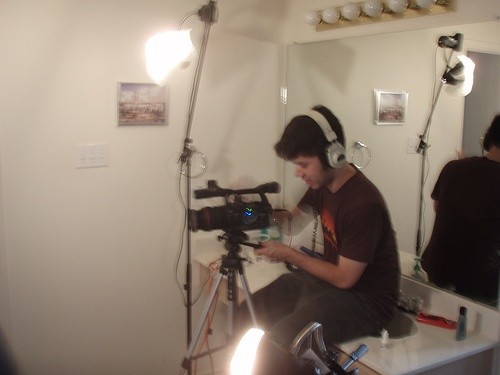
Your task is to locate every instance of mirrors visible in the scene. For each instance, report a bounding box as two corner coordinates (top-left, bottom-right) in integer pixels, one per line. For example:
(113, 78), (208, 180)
(281, 18), (500, 310)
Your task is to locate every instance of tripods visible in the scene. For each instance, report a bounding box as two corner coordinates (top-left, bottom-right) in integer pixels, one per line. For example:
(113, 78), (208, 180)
(178, 252), (262, 375)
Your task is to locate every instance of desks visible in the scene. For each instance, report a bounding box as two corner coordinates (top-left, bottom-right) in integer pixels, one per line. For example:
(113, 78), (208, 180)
(191, 249), (494, 375)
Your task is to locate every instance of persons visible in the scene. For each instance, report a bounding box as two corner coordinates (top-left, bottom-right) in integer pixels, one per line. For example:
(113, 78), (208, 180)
(420, 113), (500, 300)
(233, 105), (401, 375)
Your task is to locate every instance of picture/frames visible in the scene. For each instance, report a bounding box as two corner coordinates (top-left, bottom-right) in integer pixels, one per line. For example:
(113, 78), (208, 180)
(374, 90), (410, 127)
(115, 80), (170, 127)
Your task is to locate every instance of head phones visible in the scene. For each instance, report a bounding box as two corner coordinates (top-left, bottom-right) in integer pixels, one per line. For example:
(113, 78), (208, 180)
(305, 109), (348, 169)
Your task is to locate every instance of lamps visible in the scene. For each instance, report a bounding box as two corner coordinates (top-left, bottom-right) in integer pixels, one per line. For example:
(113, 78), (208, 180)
(229, 321), (369, 375)
(303, 0), (458, 32)
(411, 31), (478, 282)
(143, 0), (220, 375)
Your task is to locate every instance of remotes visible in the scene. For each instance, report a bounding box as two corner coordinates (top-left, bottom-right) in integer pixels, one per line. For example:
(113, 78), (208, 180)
(415, 312), (457, 330)
